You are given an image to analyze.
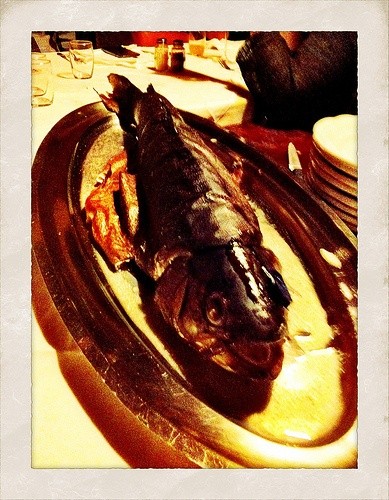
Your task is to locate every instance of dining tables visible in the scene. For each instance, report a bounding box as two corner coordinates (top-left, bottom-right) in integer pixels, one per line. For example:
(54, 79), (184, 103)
(31, 41), (358, 469)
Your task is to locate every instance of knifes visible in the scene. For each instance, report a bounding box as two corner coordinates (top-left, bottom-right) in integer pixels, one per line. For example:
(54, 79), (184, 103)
(211, 46), (231, 70)
(288, 142), (303, 180)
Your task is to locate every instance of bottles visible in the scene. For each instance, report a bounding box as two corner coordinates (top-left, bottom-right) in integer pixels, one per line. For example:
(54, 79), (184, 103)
(154, 38), (168, 71)
(171, 40), (185, 74)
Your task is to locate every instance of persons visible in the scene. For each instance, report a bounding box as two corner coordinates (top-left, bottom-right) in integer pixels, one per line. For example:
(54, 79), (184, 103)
(31, 31), (59, 52)
(236, 31), (358, 133)
(128, 31), (229, 57)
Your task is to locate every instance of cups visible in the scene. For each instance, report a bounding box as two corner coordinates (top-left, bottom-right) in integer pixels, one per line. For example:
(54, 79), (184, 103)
(69, 40), (94, 79)
(31, 52), (48, 96)
(189, 31), (206, 57)
(31, 59), (54, 106)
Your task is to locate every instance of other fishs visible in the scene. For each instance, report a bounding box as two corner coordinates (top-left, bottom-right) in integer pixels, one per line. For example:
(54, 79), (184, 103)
(80, 69), (294, 384)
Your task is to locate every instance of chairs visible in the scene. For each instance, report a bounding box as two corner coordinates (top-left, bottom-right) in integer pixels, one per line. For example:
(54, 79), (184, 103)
(127, 31), (230, 44)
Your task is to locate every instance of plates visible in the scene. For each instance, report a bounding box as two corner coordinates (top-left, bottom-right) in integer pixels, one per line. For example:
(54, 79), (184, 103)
(30, 100), (358, 469)
(307, 114), (358, 233)
